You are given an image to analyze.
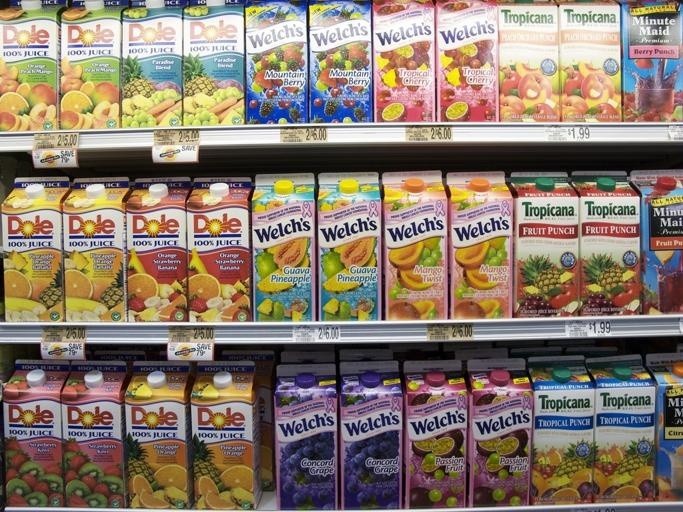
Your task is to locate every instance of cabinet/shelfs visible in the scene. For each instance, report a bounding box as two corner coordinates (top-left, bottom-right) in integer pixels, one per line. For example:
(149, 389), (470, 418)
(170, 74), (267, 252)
(1, 0), (683, 512)
